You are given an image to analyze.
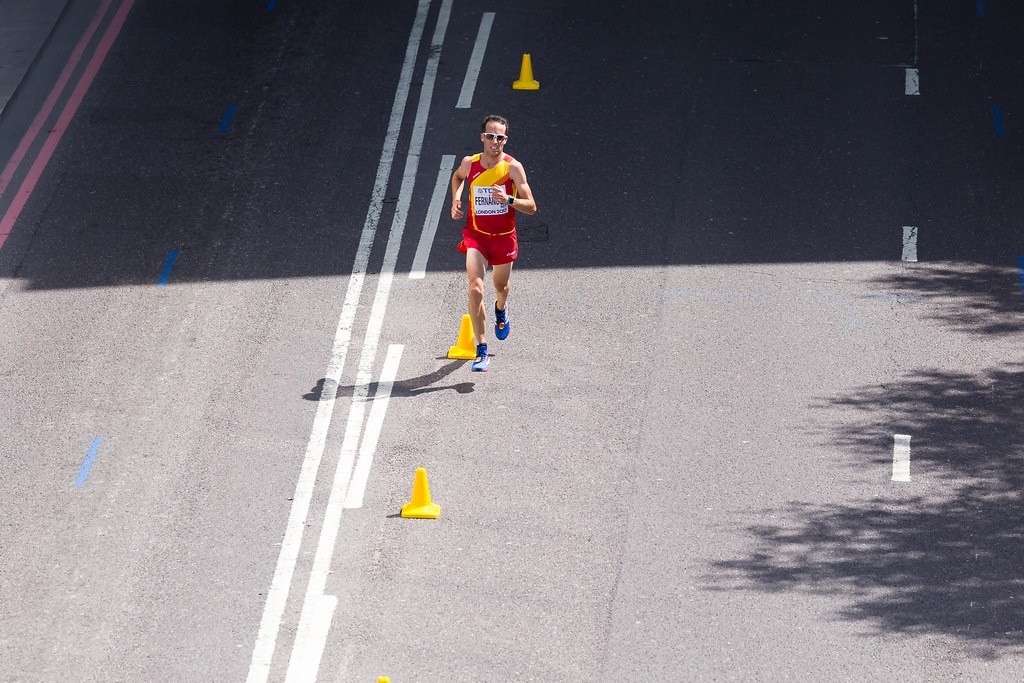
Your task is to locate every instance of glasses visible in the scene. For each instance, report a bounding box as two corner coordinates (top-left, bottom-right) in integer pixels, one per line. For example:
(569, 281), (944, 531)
(483, 133), (507, 142)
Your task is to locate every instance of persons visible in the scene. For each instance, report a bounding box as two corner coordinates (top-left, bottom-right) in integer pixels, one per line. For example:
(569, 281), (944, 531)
(451, 115), (537, 373)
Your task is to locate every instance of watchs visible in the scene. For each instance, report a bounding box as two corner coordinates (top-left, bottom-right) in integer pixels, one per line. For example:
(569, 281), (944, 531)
(507, 195), (515, 206)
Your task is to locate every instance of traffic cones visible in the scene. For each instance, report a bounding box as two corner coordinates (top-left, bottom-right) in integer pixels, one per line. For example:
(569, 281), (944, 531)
(446, 312), (480, 361)
(400, 467), (442, 520)
(511, 53), (540, 91)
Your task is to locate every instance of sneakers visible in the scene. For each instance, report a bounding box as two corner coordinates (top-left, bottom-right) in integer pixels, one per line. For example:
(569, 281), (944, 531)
(472, 346), (489, 371)
(495, 300), (510, 340)
